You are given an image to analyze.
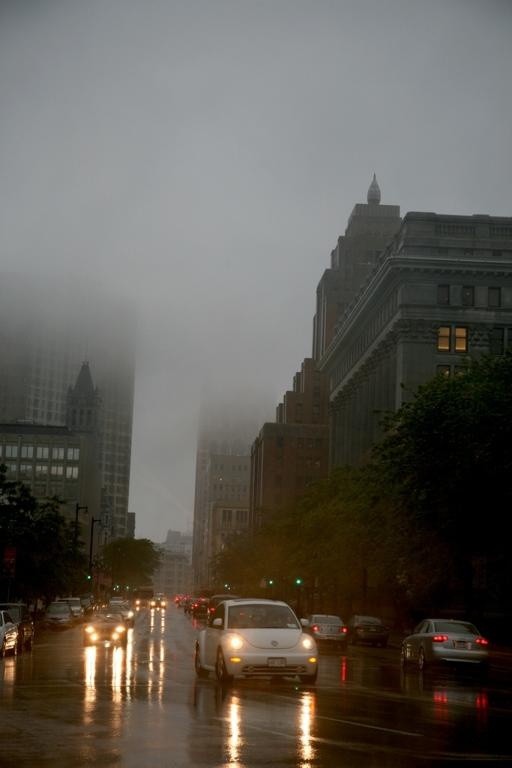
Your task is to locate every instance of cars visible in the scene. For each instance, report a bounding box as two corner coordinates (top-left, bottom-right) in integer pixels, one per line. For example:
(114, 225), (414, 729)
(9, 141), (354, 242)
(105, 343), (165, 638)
(400, 618), (489, 670)
(174, 593), (391, 684)
(0, 593), (169, 657)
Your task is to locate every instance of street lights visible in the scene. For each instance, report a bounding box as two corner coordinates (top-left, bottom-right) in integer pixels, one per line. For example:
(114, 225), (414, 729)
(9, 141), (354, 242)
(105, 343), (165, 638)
(265, 577), (303, 621)
(73, 502), (103, 569)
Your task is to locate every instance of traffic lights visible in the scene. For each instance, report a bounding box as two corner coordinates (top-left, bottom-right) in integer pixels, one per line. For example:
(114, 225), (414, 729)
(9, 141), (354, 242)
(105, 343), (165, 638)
(87, 571), (92, 581)
